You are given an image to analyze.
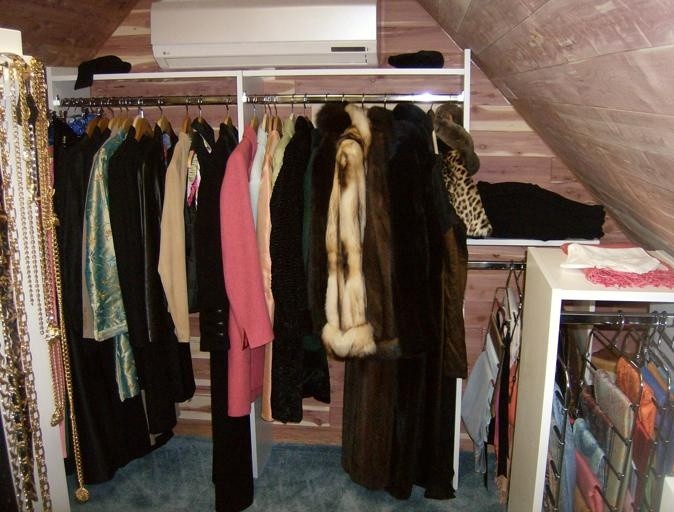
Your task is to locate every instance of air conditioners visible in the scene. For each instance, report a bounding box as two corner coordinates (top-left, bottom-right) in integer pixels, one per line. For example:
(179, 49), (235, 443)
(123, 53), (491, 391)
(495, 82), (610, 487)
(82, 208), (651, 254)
(144, 2), (384, 74)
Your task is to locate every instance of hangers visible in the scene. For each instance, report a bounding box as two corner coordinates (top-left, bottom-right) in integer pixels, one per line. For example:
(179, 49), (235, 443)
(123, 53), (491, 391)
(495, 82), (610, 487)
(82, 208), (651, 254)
(83, 92), (299, 144)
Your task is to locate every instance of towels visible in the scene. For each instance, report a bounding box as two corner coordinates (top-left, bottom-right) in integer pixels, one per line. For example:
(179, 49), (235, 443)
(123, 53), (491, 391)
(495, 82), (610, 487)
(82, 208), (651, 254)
(454, 290), (520, 484)
(545, 364), (672, 507)
